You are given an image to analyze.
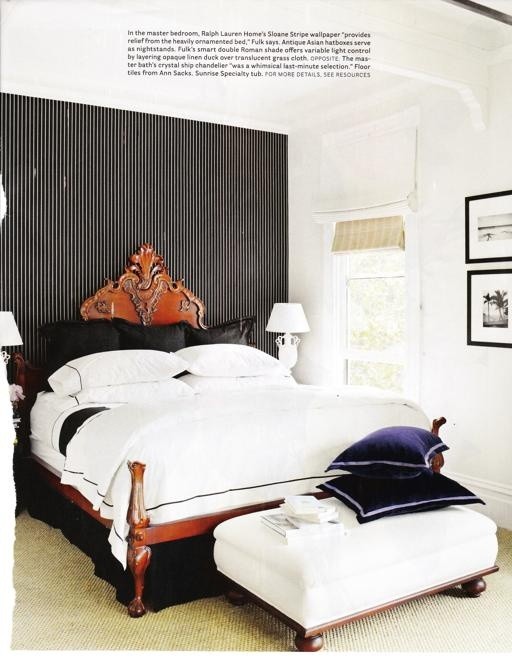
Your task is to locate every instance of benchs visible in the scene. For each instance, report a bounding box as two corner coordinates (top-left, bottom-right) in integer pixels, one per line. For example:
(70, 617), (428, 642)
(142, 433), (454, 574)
(213, 489), (500, 648)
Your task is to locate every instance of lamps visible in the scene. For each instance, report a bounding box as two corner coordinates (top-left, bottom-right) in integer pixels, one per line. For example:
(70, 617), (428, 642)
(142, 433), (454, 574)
(265, 300), (311, 368)
(0, 308), (26, 400)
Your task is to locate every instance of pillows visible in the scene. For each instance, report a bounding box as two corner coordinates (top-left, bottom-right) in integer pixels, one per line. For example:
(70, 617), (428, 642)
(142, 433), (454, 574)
(45, 347), (191, 396)
(36, 317), (118, 392)
(111, 316), (189, 348)
(321, 423), (449, 472)
(318, 474), (489, 522)
(180, 314), (259, 349)
(167, 340), (294, 380)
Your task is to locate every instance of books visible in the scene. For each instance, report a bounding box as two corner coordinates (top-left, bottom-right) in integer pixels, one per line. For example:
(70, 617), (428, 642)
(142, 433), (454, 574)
(260, 494), (341, 537)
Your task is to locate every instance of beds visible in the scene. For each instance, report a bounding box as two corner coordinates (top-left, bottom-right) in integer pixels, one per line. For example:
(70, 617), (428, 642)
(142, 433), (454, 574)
(18, 244), (448, 614)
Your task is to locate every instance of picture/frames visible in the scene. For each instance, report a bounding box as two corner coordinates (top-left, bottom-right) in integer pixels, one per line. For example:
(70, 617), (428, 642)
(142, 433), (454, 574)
(464, 269), (511, 349)
(464, 189), (512, 263)
